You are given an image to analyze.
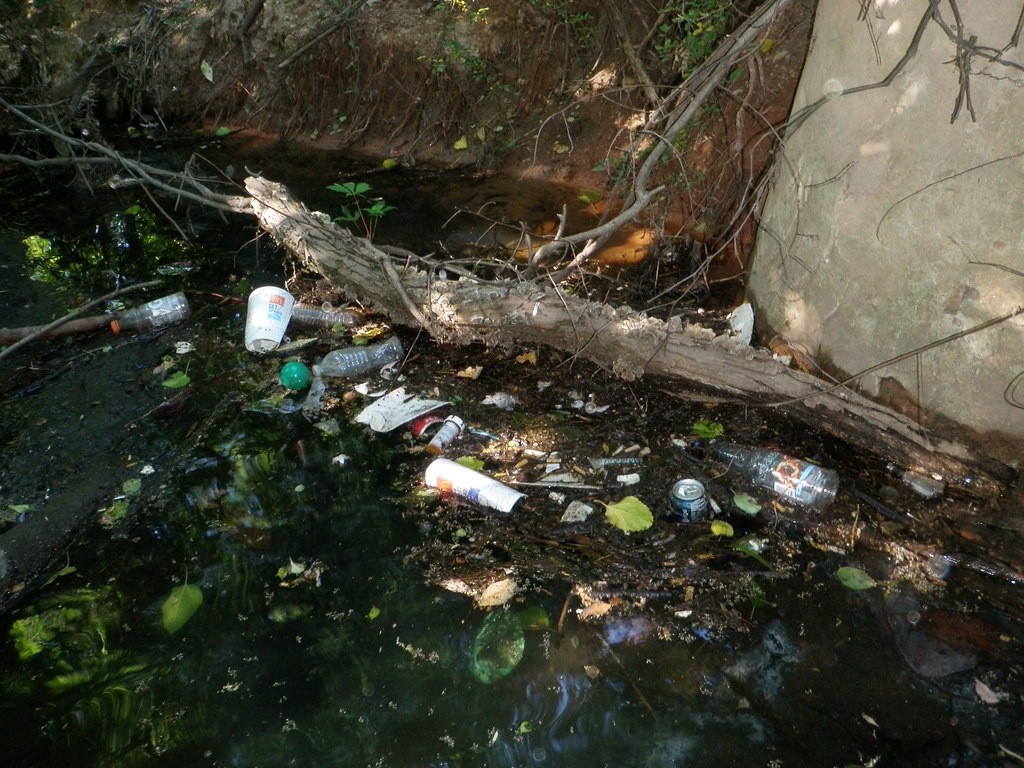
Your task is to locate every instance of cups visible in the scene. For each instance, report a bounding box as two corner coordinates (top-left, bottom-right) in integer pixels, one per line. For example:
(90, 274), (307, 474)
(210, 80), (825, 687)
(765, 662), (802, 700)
(245, 286), (296, 353)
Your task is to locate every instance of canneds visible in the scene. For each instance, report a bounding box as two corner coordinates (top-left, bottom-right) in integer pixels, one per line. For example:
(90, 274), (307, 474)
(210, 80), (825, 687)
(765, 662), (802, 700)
(666, 478), (708, 525)
(405, 413), (444, 440)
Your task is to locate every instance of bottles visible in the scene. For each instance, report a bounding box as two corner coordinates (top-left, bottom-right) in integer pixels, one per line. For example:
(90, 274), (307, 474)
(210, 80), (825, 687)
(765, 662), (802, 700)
(111, 292), (192, 335)
(311, 336), (405, 378)
(710, 440), (839, 508)
(428, 414), (464, 454)
(293, 299), (362, 327)
(885, 462), (945, 503)
